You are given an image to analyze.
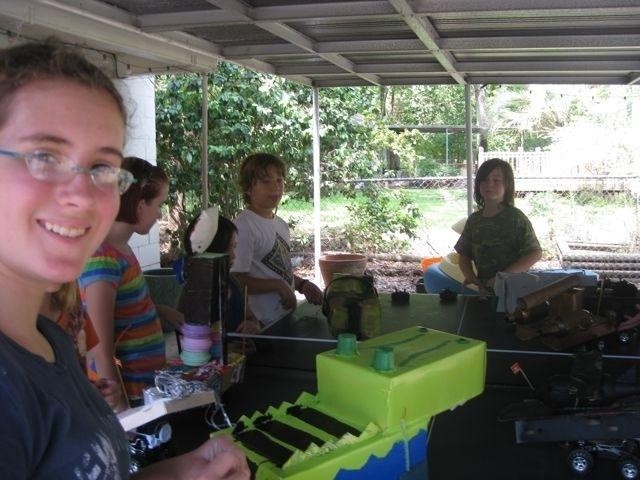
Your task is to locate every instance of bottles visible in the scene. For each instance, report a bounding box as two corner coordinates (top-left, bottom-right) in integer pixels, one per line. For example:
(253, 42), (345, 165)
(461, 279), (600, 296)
(359, 299), (380, 338)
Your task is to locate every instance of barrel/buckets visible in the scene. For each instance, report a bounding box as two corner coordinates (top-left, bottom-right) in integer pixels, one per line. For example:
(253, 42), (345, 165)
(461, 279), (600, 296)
(142, 267), (183, 334)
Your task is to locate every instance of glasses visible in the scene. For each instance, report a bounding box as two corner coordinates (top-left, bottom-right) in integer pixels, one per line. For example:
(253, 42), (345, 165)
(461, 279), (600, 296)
(0, 149), (138, 197)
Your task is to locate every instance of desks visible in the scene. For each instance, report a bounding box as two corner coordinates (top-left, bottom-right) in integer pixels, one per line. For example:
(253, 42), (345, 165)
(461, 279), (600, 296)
(131, 291), (640, 480)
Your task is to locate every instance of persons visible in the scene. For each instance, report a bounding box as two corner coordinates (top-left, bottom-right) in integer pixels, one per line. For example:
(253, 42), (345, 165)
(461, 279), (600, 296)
(453, 159), (542, 296)
(1, 44), (251, 479)
(231, 152), (323, 326)
(39, 277), (122, 414)
(183, 206), (266, 425)
(77, 157), (187, 412)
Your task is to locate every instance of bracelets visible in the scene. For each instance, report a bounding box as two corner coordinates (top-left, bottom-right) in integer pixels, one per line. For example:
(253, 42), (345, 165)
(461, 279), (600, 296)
(298, 278), (308, 295)
